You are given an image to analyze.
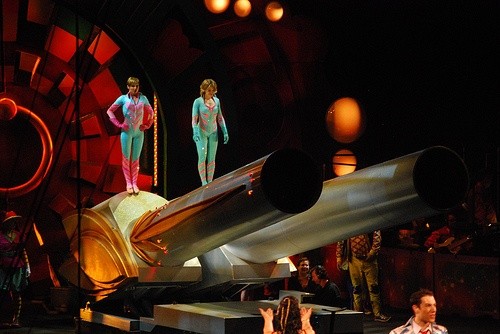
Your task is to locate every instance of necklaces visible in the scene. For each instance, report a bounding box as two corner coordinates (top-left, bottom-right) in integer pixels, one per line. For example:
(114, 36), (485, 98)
(208, 100), (214, 104)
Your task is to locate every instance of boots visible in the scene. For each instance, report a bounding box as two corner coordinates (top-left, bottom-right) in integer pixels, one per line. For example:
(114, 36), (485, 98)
(11, 289), (23, 327)
(0, 292), (10, 327)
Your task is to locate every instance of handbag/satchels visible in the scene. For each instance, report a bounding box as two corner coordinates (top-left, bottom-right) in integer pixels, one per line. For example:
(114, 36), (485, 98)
(340, 259), (349, 271)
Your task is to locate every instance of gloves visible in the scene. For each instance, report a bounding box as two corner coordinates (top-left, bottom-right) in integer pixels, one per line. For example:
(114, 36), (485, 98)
(25, 266), (31, 278)
(220, 124), (229, 144)
(139, 119), (154, 130)
(193, 126), (201, 142)
(110, 117), (129, 131)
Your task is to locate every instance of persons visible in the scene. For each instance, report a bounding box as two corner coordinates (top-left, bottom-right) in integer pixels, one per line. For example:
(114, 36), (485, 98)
(259, 295), (315, 334)
(106, 76), (156, 195)
(288, 256), (317, 305)
(388, 289), (448, 334)
(336, 229), (392, 324)
(396, 181), (499, 260)
(192, 78), (230, 187)
(310, 265), (342, 308)
(0, 210), (31, 326)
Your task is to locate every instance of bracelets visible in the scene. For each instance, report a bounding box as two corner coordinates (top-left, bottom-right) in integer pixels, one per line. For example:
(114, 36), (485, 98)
(301, 318), (310, 322)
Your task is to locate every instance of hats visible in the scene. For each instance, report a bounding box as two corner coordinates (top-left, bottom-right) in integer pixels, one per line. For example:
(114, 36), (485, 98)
(2, 211), (22, 223)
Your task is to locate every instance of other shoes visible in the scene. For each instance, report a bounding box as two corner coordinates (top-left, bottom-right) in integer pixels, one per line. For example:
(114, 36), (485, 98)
(132, 185), (140, 193)
(126, 186), (133, 194)
(374, 313), (392, 323)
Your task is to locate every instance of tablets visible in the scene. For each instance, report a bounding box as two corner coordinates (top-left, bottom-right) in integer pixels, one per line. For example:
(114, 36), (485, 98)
(315, 265), (325, 279)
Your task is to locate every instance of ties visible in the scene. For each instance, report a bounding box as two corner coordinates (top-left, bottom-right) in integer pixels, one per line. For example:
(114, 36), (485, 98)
(419, 330), (429, 334)
